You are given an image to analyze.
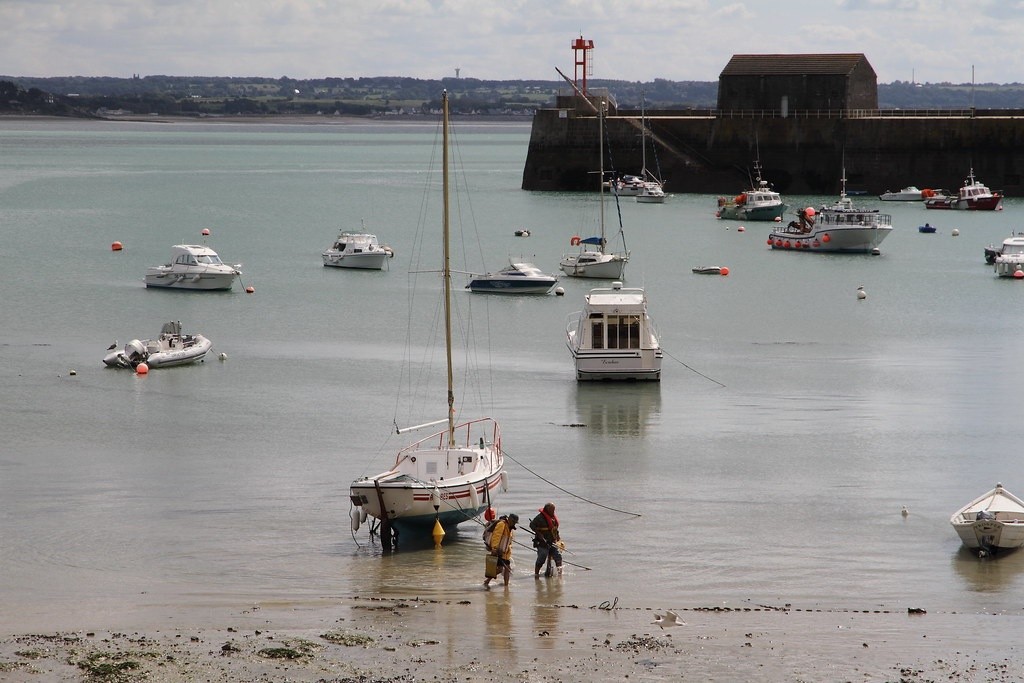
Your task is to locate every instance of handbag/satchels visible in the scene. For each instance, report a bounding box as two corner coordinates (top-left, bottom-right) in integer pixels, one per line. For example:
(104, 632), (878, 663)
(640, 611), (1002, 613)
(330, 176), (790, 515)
(485, 553), (498, 579)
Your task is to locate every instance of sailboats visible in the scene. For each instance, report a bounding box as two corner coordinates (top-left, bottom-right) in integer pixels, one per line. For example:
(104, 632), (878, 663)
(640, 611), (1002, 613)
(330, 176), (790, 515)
(602, 89), (666, 197)
(341, 82), (508, 543)
(559, 94), (633, 278)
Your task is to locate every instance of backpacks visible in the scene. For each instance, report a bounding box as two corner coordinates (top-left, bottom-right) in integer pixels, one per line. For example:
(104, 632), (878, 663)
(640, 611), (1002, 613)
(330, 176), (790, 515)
(483, 516), (508, 551)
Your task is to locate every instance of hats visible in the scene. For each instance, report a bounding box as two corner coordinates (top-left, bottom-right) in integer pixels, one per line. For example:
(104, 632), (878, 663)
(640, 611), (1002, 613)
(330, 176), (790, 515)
(510, 514), (519, 524)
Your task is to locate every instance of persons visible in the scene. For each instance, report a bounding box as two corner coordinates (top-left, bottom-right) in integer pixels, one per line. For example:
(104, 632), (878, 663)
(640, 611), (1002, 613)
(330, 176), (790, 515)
(530, 503), (563, 574)
(484, 514), (518, 586)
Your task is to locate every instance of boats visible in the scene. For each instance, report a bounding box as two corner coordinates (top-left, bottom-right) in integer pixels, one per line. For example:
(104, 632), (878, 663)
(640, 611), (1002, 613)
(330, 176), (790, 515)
(142, 244), (244, 291)
(691, 266), (721, 276)
(634, 181), (666, 203)
(715, 158), (789, 221)
(563, 281), (664, 382)
(923, 167), (1004, 210)
(766, 142), (894, 254)
(320, 217), (395, 271)
(996, 230), (1024, 279)
(949, 481), (1024, 559)
(878, 185), (943, 201)
(102, 320), (213, 371)
(465, 262), (560, 296)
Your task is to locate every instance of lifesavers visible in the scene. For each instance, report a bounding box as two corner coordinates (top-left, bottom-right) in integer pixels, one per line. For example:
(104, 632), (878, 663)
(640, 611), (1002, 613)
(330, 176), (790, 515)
(570, 236), (581, 247)
